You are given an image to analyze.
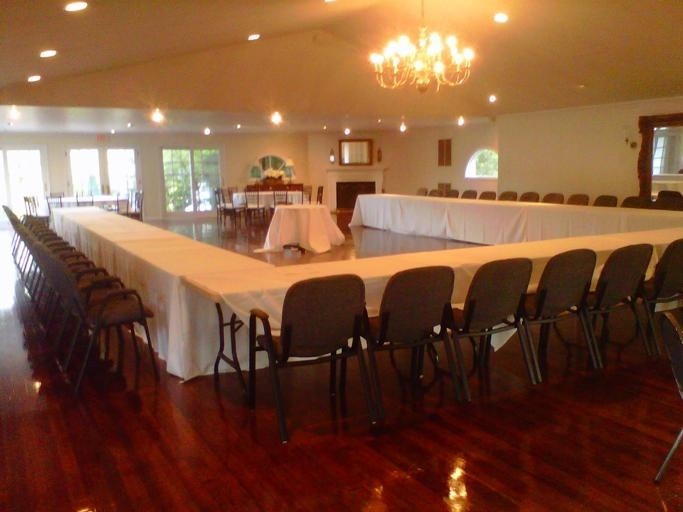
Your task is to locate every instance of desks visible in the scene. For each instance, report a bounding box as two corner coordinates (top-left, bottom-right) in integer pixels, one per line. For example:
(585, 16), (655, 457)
(48, 206), (274, 380)
(254, 203), (346, 258)
(347, 192), (682, 247)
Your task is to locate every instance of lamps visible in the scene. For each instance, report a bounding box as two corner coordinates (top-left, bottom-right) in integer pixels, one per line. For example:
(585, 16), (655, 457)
(367, 1), (475, 97)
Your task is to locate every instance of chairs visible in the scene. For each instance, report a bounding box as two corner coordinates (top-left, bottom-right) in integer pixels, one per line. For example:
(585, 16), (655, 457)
(211, 181), (327, 226)
(640, 237), (682, 376)
(524, 247), (601, 382)
(367, 265), (462, 403)
(447, 255), (537, 386)
(417, 186), (682, 211)
(241, 273), (382, 445)
(649, 310), (682, 486)
(2, 204), (161, 401)
(586, 243), (655, 368)
(21, 188), (147, 222)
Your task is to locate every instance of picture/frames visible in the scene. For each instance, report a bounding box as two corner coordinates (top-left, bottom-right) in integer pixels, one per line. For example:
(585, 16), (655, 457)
(337, 138), (374, 166)
